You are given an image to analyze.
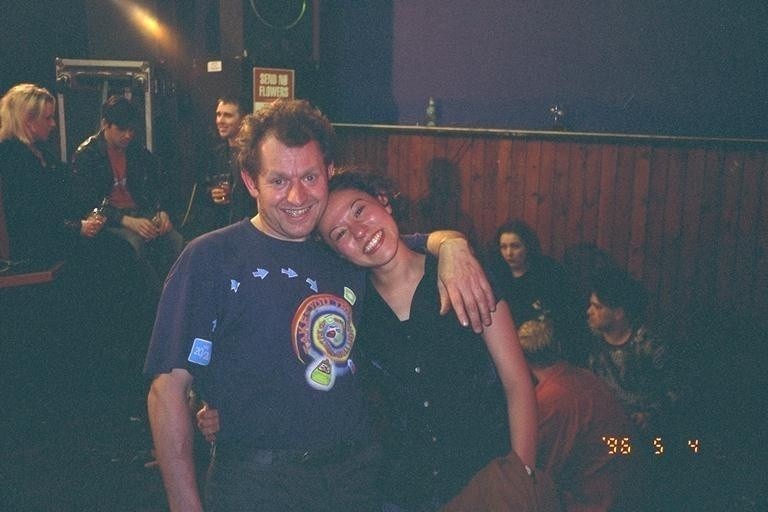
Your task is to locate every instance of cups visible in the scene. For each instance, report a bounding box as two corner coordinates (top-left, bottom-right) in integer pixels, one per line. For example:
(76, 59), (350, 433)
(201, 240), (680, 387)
(218, 172), (232, 204)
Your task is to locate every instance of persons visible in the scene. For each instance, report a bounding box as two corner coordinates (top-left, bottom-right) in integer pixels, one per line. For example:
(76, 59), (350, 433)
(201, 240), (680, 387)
(0, 80), (186, 264)
(519, 321), (637, 512)
(587, 271), (684, 375)
(204, 95), (246, 219)
(188, 162), (535, 512)
(143, 98), (497, 511)
(488, 220), (572, 357)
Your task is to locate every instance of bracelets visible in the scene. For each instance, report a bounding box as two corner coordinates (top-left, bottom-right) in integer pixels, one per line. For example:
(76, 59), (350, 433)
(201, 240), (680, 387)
(438, 234), (472, 257)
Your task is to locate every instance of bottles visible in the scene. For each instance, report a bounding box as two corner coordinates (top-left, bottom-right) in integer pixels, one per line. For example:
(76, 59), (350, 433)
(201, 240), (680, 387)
(86, 196), (111, 235)
(425, 97), (437, 126)
